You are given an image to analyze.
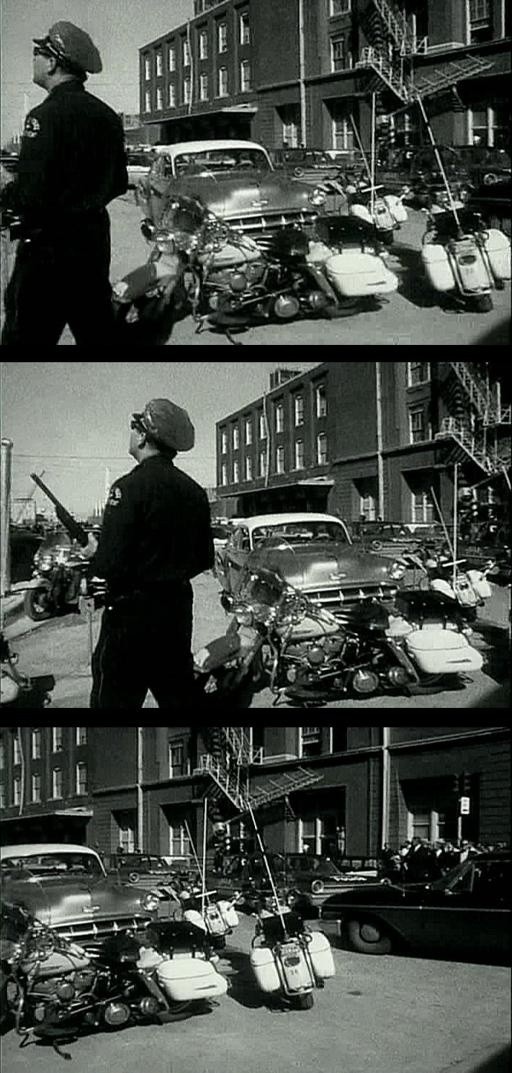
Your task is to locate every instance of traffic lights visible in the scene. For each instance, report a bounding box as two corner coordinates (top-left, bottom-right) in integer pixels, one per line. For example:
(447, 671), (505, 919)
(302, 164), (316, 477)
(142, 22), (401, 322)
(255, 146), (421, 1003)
(450, 770), (460, 793)
(463, 769), (472, 794)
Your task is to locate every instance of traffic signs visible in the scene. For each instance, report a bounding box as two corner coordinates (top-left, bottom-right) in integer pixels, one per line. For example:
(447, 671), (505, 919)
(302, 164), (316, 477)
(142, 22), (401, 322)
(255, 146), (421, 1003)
(461, 796), (471, 814)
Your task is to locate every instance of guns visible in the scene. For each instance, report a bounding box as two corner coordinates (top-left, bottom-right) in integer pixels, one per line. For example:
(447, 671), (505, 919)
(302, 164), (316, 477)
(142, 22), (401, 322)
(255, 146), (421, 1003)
(30, 472), (108, 609)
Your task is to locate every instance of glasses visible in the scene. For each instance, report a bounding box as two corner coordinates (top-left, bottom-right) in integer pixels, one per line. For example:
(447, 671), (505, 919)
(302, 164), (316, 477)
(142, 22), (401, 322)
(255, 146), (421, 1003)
(130, 420), (142, 436)
(31, 46), (53, 60)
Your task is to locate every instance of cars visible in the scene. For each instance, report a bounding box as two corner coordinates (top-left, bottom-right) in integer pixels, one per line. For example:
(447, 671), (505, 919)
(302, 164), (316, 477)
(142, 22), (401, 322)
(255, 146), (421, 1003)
(317, 850), (510, 958)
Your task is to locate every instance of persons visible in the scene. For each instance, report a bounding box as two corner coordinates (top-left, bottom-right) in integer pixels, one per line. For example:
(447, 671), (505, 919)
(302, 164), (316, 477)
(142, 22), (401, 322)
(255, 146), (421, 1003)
(375, 135), (389, 167)
(212, 841), (223, 873)
(378, 834), (509, 882)
(0, 17), (128, 347)
(457, 508), (472, 542)
(68, 392), (214, 707)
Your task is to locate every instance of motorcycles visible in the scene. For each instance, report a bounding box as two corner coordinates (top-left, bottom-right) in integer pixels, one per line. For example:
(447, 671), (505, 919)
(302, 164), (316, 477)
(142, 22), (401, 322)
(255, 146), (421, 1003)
(22, 526), (102, 621)
(191, 508), (494, 717)
(1, 820), (381, 1054)
(106, 138), (512, 345)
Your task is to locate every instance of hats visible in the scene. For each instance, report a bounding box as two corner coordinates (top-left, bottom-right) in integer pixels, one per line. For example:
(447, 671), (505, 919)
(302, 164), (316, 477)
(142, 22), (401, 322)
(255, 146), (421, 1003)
(132, 398), (195, 450)
(32, 21), (101, 74)
